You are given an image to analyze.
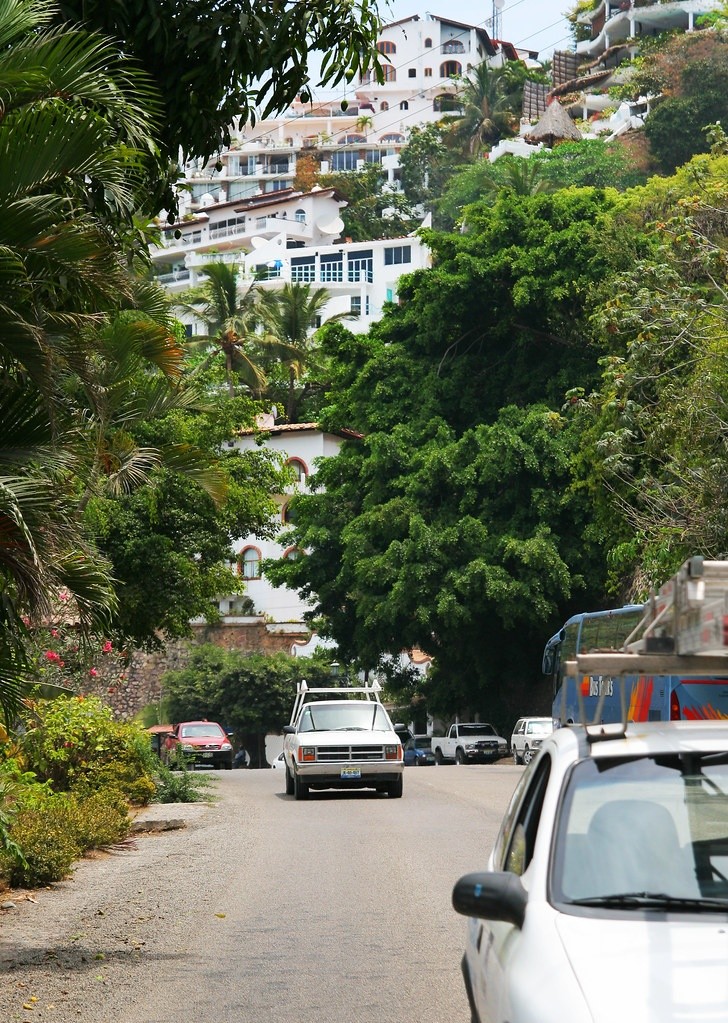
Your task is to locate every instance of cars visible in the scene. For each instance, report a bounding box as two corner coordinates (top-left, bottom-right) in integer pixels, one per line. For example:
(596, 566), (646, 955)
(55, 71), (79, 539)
(271, 678), (435, 800)
(162, 721), (251, 771)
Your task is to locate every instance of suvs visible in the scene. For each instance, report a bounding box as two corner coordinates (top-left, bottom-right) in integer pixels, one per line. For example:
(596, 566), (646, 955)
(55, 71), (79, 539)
(511, 716), (552, 765)
(452, 655), (728, 1023)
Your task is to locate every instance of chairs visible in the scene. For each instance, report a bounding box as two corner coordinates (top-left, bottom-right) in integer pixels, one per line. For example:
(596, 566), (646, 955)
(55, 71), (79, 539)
(588, 799), (689, 898)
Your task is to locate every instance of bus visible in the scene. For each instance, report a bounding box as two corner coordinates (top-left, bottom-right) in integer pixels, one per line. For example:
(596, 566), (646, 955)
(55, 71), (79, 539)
(541, 604), (728, 731)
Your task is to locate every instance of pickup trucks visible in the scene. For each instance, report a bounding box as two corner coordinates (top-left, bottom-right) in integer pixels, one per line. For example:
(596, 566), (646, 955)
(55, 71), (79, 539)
(431, 723), (508, 766)
